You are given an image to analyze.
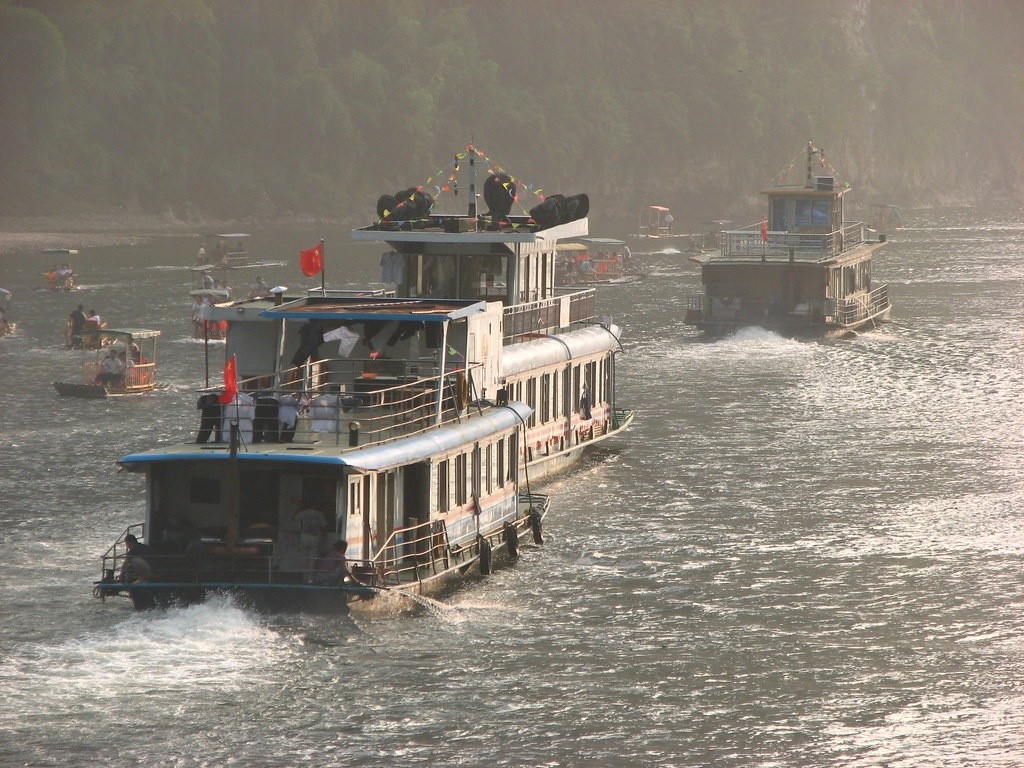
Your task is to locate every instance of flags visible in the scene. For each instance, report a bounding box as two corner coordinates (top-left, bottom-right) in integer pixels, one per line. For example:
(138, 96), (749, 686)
(216, 355), (237, 404)
(301, 243), (322, 276)
(761, 216), (768, 241)
(378, 144), (545, 228)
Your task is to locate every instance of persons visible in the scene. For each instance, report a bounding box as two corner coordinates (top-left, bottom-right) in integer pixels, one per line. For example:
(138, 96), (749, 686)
(255, 275), (270, 291)
(556, 248), (621, 278)
(192, 275), (232, 334)
(197, 242), (207, 265)
(665, 212), (674, 233)
(69, 305), (146, 388)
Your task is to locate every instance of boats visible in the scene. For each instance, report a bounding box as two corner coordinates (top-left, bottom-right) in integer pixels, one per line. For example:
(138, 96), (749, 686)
(0, 288), (17, 339)
(188, 288), (233, 340)
(681, 139), (893, 344)
(200, 133), (633, 489)
(53, 327), (160, 398)
(686, 219), (746, 267)
(65, 319), (108, 349)
(191, 230), (251, 271)
(40, 263), (80, 292)
(626, 205), (716, 248)
(91, 239), (549, 624)
(554, 237), (646, 290)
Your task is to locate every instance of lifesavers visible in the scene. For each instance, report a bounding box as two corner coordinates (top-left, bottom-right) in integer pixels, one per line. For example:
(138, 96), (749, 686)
(481, 542), (493, 575)
(532, 510), (544, 545)
(457, 372), (468, 410)
(507, 523), (519, 559)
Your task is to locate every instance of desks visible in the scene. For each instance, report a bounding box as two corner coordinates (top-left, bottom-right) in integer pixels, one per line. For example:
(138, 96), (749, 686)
(395, 387), (433, 416)
